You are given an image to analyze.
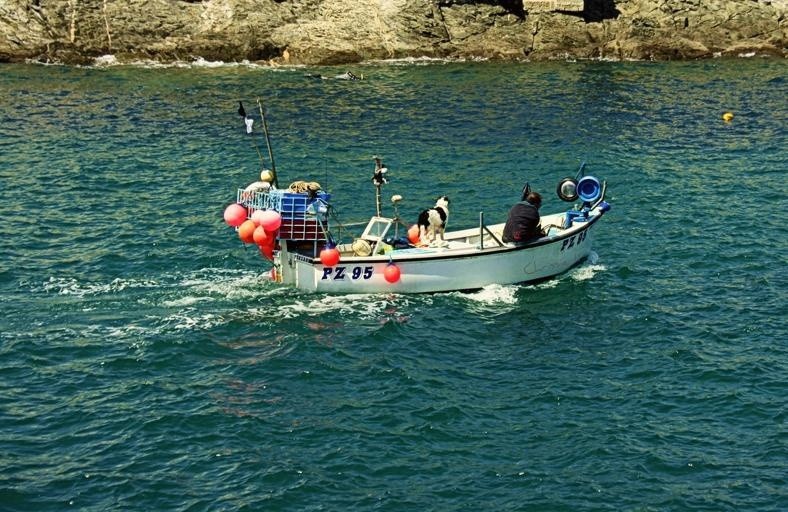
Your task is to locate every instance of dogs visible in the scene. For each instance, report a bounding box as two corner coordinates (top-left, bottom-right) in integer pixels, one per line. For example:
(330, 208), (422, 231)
(418, 195), (451, 245)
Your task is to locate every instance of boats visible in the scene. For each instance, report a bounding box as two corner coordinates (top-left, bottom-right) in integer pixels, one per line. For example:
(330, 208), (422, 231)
(237, 163), (611, 293)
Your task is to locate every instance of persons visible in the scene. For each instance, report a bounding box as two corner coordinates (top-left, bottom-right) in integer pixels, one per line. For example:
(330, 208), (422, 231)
(502, 193), (544, 248)
